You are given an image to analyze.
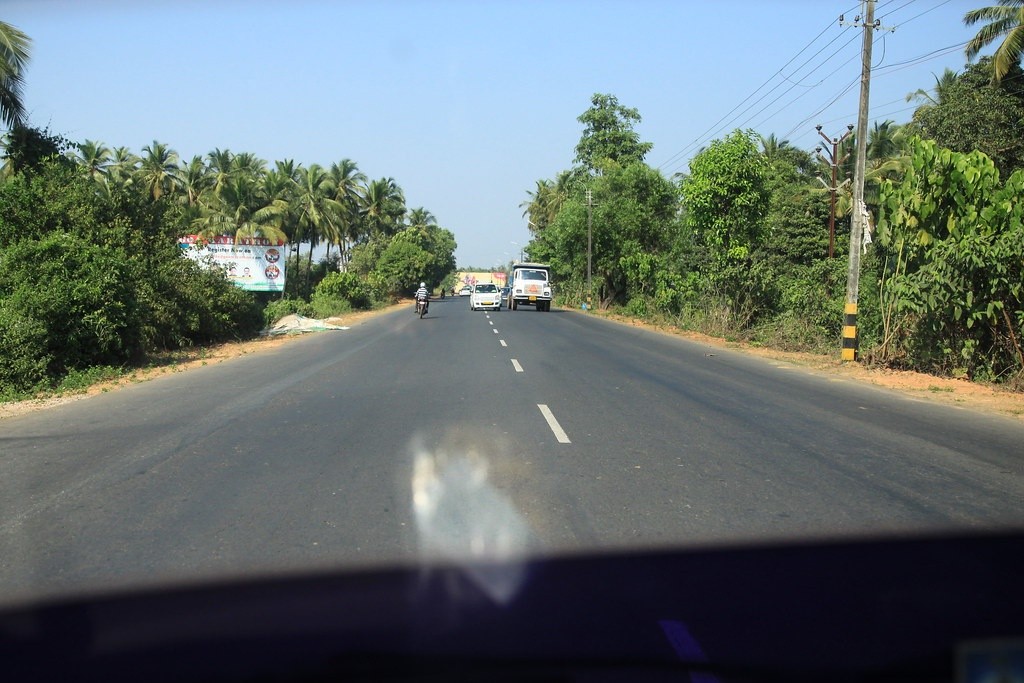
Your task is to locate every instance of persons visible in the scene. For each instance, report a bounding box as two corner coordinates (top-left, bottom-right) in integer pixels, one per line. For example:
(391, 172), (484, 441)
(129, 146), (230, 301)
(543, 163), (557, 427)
(229, 266), (250, 277)
(441, 288), (445, 296)
(451, 286), (455, 292)
(414, 282), (430, 312)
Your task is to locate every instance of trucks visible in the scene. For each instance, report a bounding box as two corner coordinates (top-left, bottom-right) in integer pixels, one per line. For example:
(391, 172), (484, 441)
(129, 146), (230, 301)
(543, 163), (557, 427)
(507, 262), (552, 311)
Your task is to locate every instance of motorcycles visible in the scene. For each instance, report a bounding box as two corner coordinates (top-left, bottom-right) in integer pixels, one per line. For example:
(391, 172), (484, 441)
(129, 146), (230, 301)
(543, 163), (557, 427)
(414, 292), (432, 319)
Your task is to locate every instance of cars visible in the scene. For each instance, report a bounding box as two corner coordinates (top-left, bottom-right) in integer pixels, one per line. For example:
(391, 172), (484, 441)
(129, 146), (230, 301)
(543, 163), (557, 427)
(470, 283), (502, 311)
(496, 285), (512, 307)
(459, 285), (474, 297)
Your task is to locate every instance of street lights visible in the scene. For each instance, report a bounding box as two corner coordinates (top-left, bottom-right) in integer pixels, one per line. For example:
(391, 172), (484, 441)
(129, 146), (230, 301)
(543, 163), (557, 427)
(510, 241), (523, 263)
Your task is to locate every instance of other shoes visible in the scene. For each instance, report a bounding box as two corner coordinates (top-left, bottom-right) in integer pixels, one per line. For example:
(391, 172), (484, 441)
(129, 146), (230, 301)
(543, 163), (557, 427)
(414, 310), (417, 313)
(426, 308), (428, 313)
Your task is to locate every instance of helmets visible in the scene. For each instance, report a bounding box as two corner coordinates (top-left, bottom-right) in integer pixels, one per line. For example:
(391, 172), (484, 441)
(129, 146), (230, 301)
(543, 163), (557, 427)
(420, 282), (425, 287)
